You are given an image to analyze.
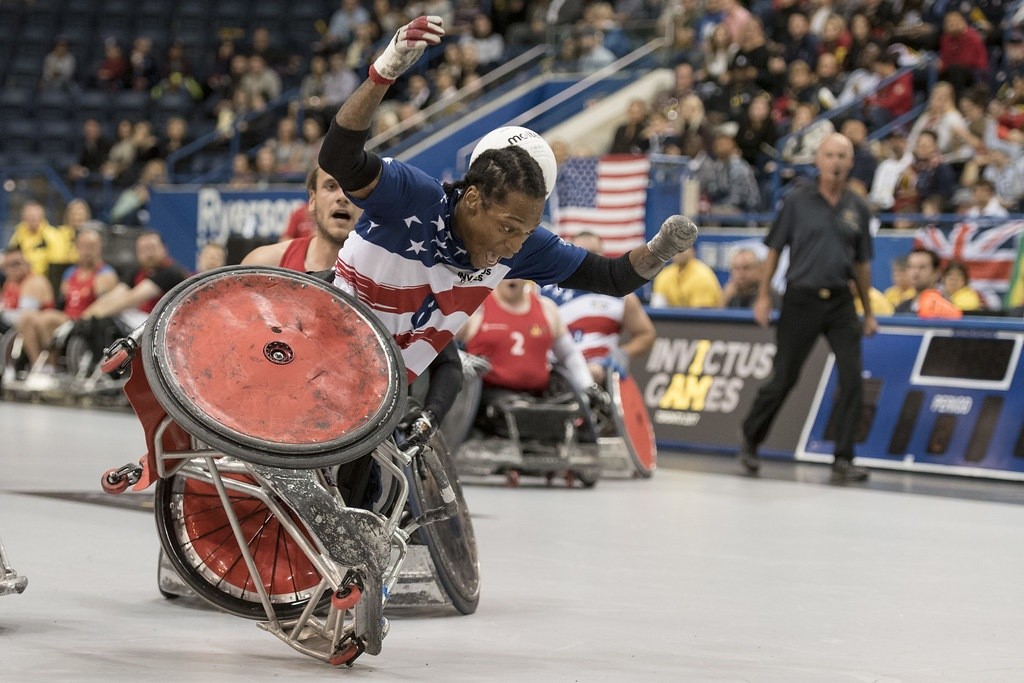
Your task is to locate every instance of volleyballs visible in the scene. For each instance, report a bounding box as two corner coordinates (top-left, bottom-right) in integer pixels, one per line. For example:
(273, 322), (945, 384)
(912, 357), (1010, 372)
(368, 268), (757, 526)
(468, 127), (557, 202)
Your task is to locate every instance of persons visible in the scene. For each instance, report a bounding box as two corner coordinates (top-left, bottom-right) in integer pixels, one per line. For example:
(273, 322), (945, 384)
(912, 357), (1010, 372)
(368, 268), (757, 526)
(39, 26), (309, 191)
(609, 1), (1024, 236)
(231, 0), (628, 190)
(239, 162), (366, 277)
(736, 134), (880, 485)
(316, 15), (699, 382)
(845, 247), (982, 317)
(721, 238), (784, 310)
(0, 198), (231, 390)
(542, 232), (656, 381)
(454, 277), (596, 443)
(649, 245), (727, 310)
(275, 200), (318, 241)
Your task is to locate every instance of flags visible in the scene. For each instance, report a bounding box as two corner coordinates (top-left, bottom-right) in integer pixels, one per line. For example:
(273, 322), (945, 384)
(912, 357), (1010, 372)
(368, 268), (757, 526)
(548, 156), (651, 259)
(911, 219), (1024, 313)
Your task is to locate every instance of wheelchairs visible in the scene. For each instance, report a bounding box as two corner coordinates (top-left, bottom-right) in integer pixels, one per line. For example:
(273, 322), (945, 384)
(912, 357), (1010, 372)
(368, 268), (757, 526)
(477, 349), (660, 487)
(0, 325), (112, 405)
(107, 263), (484, 666)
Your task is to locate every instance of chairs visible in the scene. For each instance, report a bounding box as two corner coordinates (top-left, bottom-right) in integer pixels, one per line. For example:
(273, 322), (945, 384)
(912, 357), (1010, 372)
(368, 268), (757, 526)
(0, 0), (336, 225)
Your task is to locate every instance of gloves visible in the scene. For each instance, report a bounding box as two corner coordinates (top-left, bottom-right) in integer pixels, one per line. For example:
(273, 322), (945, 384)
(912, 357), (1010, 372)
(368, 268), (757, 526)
(369, 15), (445, 85)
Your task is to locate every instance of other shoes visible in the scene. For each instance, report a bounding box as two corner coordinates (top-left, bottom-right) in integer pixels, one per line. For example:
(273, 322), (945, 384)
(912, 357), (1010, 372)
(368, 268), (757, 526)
(739, 430), (759, 473)
(832, 457), (868, 481)
